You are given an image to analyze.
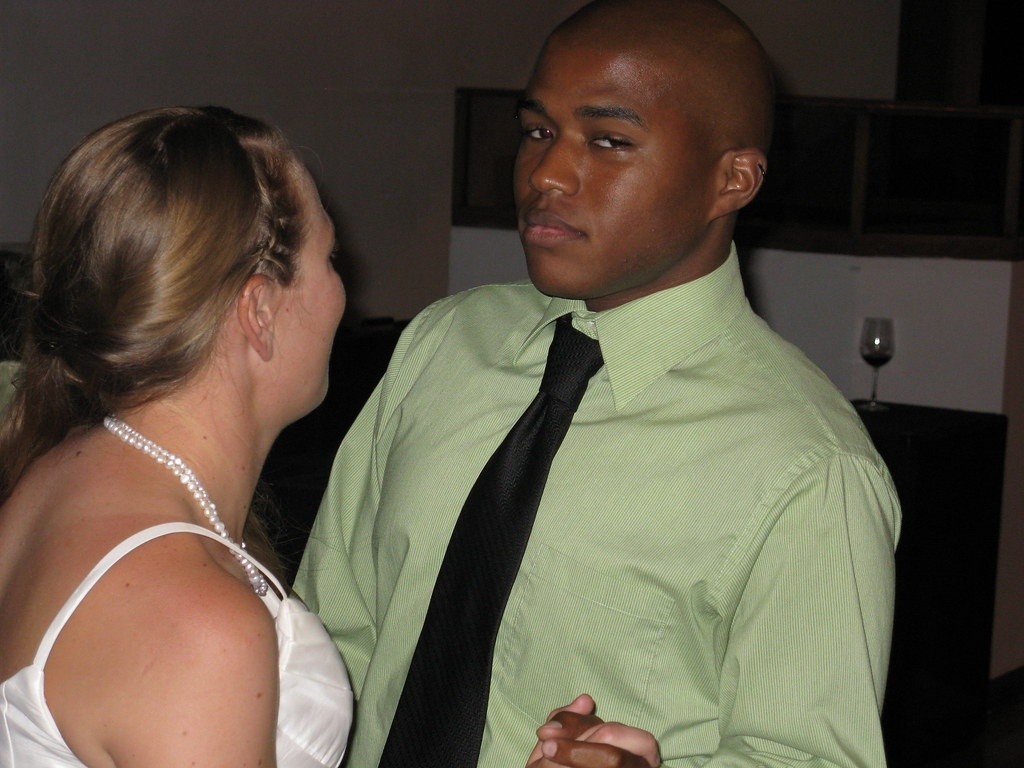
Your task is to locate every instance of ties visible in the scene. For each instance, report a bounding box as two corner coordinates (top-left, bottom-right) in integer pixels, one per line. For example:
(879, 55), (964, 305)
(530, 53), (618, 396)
(378, 312), (604, 768)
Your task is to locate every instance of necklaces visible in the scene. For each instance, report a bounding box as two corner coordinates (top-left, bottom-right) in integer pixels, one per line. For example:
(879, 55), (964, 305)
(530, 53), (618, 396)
(104, 413), (268, 596)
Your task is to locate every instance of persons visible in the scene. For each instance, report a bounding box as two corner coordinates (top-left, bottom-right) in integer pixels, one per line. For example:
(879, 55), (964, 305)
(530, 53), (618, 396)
(295, 0), (903, 768)
(0, 107), (665, 768)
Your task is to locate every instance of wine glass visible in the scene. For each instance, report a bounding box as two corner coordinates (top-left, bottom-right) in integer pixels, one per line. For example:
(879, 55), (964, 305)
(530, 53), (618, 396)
(855, 316), (896, 411)
